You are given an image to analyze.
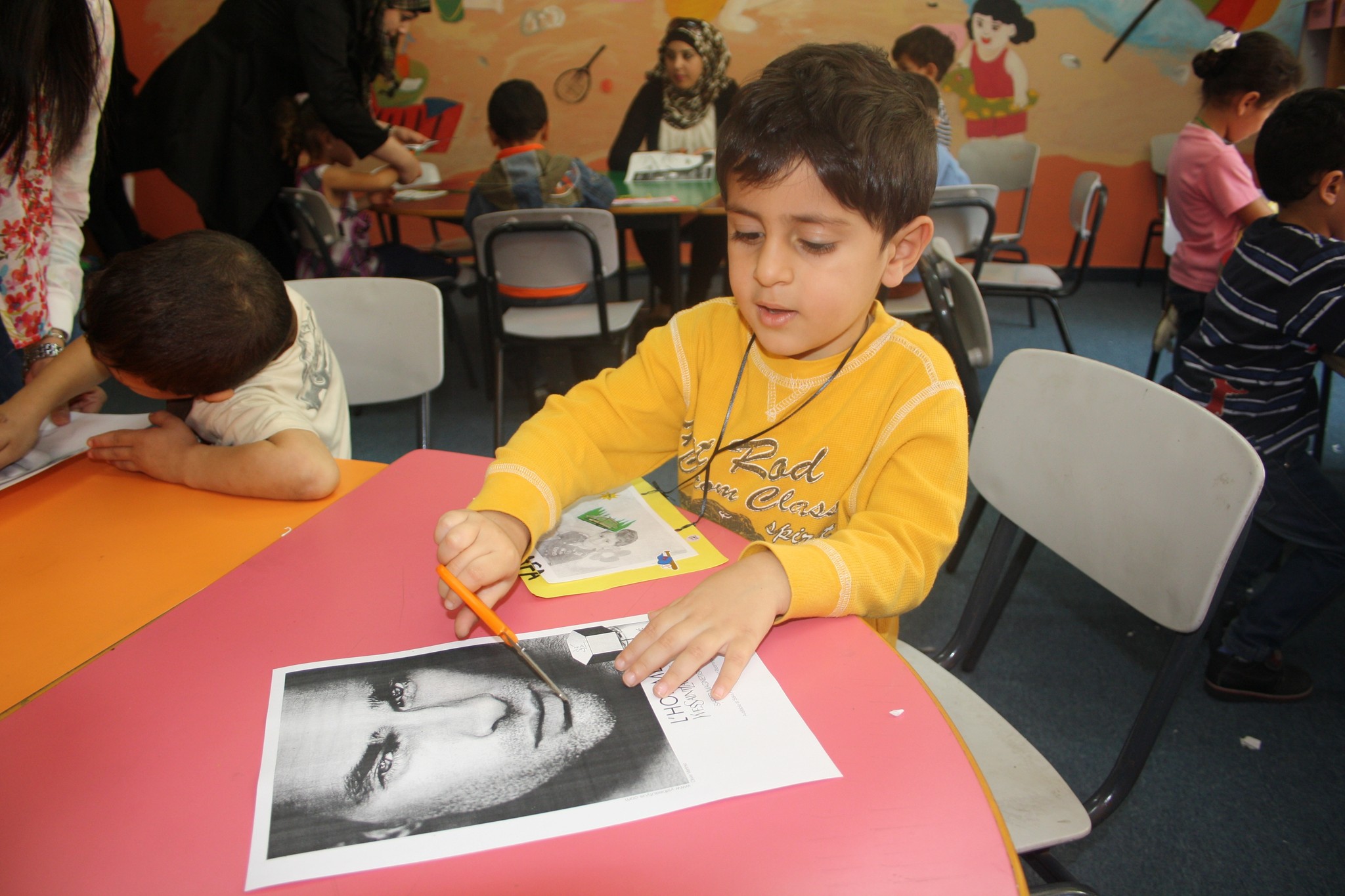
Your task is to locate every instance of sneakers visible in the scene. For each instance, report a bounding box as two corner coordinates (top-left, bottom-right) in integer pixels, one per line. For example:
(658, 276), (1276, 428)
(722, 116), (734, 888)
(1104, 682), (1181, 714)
(1203, 649), (1315, 703)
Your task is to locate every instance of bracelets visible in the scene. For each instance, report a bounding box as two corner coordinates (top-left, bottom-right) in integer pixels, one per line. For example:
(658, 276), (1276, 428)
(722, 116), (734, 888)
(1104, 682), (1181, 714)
(383, 123), (393, 136)
(21, 332), (67, 377)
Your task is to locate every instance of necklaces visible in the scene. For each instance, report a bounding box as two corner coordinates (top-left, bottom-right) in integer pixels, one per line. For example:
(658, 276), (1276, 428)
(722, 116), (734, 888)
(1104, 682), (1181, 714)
(1193, 115), (1211, 130)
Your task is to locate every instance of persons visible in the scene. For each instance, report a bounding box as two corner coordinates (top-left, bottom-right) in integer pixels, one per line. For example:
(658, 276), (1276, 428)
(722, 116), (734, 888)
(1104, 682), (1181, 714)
(887, 68), (972, 189)
(464, 77), (620, 307)
(268, 630), (631, 843)
(1166, 27), (1304, 372)
(1166, 87), (1345, 704)
(431, 43), (971, 703)
(2, 0), (115, 433)
(1, 230), (352, 500)
(891, 25), (957, 149)
(281, 94), (459, 299)
(124, 0), (435, 280)
(606, 18), (743, 330)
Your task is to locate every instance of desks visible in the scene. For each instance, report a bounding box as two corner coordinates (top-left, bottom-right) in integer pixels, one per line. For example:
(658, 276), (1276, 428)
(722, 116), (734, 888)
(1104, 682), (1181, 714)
(0, 445), (1029, 896)
(378, 165), (725, 313)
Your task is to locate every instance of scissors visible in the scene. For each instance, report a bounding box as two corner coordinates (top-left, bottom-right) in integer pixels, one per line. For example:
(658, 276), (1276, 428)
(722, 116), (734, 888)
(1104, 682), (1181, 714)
(434, 561), (572, 705)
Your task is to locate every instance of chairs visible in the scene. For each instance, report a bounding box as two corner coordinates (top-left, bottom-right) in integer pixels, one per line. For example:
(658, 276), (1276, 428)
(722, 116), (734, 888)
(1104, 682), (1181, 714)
(875, 183), (1000, 338)
(282, 185), (476, 387)
(1146, 195), (1184, 382)
(1137, 131), (1183, 318)
(281, 274), (446, 464)
(893, 346), (1268, 895)
(465, 204), (645, 420)
(963, 165), (1108, 362)
(914, 240), (1041, 669)
(956, 135), (1041, 329)
